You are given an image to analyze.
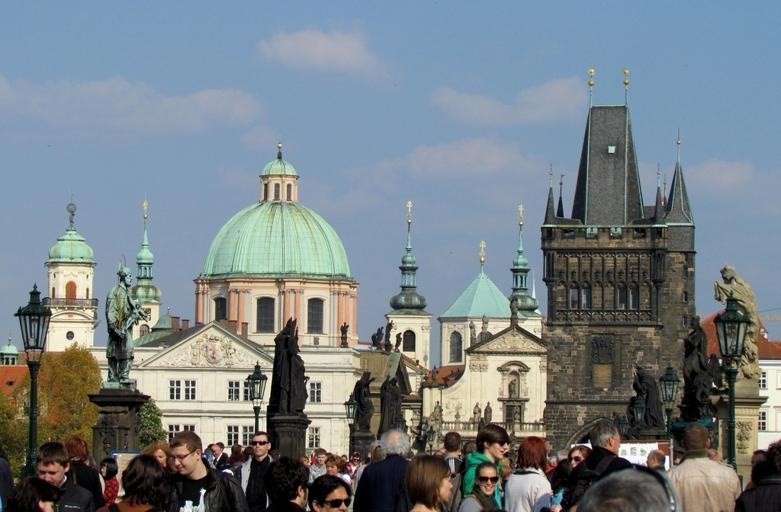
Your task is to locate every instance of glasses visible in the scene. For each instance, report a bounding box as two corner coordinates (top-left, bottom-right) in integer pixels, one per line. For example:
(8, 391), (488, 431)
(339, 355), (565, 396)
(568, 456), (581, 462)
(169, 448), (197, 462)
(477, 477), (499, 485)
(498, 440), (510, 447)
(252, 441), (269, 446)
(591, 463), (676, 511)
(324, 498), (351, 508)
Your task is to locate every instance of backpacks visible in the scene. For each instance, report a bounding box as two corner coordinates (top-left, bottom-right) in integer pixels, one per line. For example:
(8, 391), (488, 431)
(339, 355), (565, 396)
(568, 453), (617, 508)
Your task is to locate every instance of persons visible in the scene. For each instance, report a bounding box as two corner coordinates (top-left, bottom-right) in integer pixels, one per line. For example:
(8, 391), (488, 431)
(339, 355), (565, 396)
(682, 315), (708, 399)
(273, 317), (309, 415)
(104, 265), (149, 384)
(713, 265), (760, 360)
(354, 371), (374, 431)
(632, 361), (662, 420)
(380, 374), (403, 429)
(432, 401), (444, 420)
(473, 401), (493, 423)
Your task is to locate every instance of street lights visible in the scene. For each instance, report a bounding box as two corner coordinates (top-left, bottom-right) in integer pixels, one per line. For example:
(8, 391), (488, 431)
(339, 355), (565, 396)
(13, 282), (54, 478)
(619, 414), (629, 440)
(344, 392), (360, 462)
(426, 425), (437, 455)
(713, 290), (752, 493)
(658, 362), (682, 439)
(245, 360), (269, 434)
